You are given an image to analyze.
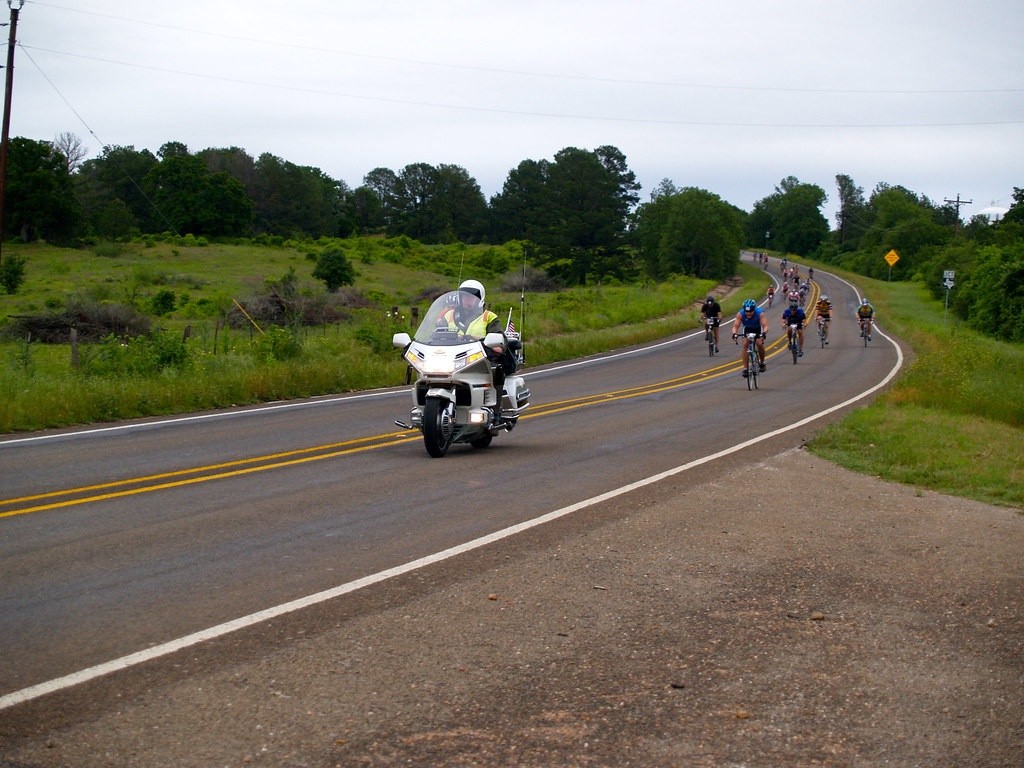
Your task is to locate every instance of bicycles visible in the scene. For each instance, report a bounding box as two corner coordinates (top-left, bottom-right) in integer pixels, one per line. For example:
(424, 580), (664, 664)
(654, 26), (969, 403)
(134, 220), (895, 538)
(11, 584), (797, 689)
(815, 317), (833, 349)
(858, 318), (874, 347)
(732, 333), (767, 391)
(699, 316), (721, 357)
(753, 256), (813, 308)
(784, 323), (804, 365)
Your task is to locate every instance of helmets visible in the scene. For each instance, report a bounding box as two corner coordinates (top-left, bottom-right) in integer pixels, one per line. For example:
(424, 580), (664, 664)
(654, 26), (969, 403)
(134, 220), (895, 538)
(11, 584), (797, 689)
(789, 302), (798, 310)
(743, 299), (756, 311)
(820, 295), (828, 301)
(862, 298), (868, 307)
(706, 296), (714, 304)
(457, 279), (486, 308)
(769, 257), (813, 293)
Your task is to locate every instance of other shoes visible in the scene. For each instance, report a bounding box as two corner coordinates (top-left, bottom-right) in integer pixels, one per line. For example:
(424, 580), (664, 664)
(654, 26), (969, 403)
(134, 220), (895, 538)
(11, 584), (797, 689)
(759, 361), (766, 373)
(742, 369), (748, 378)
(705, 334), (709, 341)
(817, 329), (820, 335)
(715, 346), (719, 352)
(825, 338), (829, 344)
(798, 350), (803, 357)
(788, 342), (792, 349)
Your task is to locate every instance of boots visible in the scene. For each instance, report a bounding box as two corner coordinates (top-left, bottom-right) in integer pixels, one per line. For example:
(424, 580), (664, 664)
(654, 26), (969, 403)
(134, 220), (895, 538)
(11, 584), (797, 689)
(492, 384), (502, 426)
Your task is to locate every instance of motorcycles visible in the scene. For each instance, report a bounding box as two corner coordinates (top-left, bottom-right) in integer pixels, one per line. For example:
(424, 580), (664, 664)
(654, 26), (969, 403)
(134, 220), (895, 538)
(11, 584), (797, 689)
(393, 290), (530, 459)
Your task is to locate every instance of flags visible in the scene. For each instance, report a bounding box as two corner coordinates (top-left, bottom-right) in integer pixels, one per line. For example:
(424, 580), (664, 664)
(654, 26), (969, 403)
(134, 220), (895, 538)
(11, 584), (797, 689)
(508, 320), (515, 337)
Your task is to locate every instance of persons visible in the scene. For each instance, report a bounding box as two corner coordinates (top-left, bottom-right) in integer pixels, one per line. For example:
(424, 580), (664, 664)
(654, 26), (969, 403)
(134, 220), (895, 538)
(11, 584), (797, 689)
(417, 279), (508, 437)
(753, 251), (768, 268)
(782, 303), (808, 357)
(701, 296), (722, 352)
(767, 285), (775, 304)
(856, 299), (874, 342)
(780, 258), (813, 306)
(815, 295), (832, 344)
(732, 299), (768, 377)
